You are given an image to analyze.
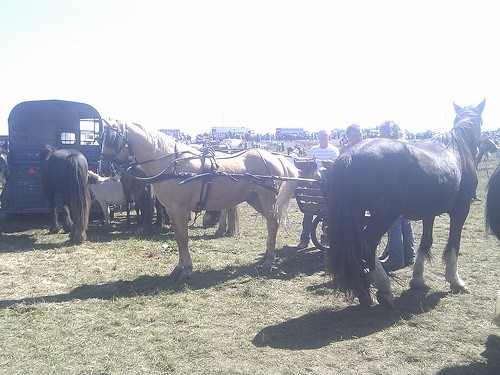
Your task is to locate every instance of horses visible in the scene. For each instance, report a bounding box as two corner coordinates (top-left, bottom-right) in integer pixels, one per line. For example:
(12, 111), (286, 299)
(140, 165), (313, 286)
(327, 97), (487, 310)
(89, 116), (299, 291)
(41, 145), (89, 244)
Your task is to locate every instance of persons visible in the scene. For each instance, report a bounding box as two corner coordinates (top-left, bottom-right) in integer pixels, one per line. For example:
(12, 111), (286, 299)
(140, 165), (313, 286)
(379, 119), (415, 271)
(298, 131), (343, 248)
(152, 122), (499, 203)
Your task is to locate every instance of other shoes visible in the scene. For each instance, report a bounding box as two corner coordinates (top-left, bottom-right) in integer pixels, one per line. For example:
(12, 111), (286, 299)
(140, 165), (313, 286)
(297, 241), (308, 248)
(405, 257), (415, 264)
(380, 258), (404, 268)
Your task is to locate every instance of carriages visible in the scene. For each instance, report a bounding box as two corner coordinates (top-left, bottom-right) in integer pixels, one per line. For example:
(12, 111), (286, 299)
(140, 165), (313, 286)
(100, 118), (390, 280)
